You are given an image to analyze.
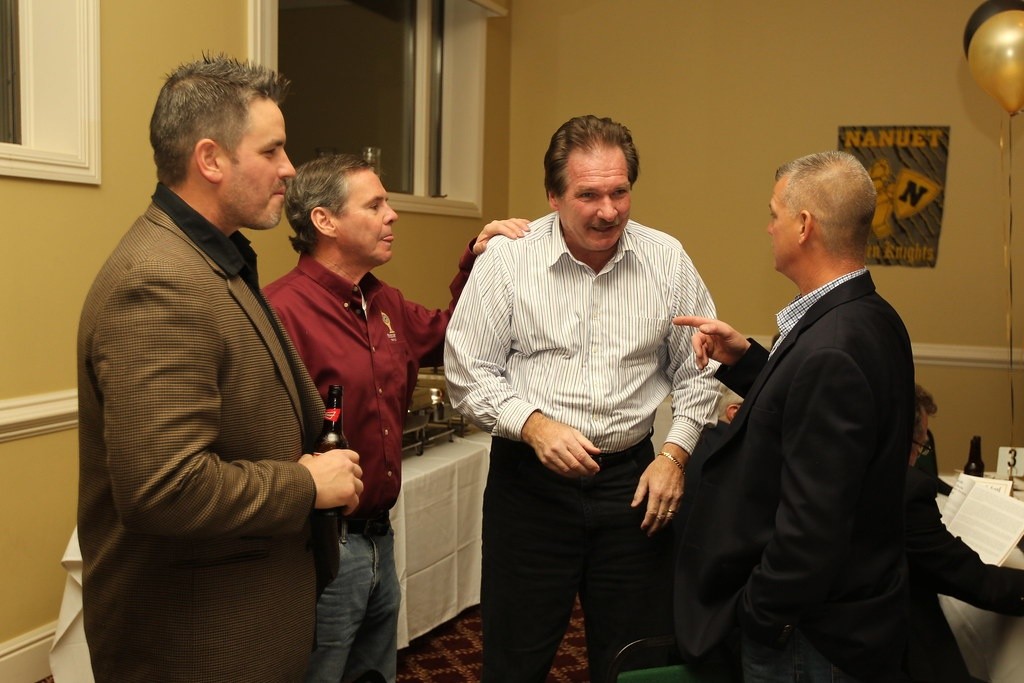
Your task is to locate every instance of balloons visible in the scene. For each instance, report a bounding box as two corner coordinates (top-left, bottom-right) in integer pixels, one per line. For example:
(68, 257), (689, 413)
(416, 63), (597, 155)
(959, 0), (1024, 121)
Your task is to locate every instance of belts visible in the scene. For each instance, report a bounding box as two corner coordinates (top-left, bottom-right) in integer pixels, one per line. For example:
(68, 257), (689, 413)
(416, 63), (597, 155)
(490, 430), (650, 469)
(337, 510), (394, 537)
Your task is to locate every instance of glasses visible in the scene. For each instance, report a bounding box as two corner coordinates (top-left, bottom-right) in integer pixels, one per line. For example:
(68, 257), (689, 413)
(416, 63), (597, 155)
(912, 439), (933, 456)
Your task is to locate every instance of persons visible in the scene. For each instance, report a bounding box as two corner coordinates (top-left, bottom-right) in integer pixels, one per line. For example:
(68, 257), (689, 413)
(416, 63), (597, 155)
(259, 149), (532, 683)
(672, 148), (1024, 683)
(77, 48), (363, 683)
(442, 115), (731, 683)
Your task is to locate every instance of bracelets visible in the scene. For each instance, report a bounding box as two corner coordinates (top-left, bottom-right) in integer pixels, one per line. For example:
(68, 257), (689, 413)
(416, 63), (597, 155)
(657, 450), (687, 476)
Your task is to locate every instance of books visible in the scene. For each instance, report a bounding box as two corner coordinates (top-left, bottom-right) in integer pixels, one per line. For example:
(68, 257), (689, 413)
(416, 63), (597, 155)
(938, 471), (1024, 567)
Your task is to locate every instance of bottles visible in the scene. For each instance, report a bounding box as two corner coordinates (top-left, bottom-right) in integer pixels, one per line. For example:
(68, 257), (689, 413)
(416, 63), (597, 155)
(313, 385), (349, 517)
(964, 435), (984, 477)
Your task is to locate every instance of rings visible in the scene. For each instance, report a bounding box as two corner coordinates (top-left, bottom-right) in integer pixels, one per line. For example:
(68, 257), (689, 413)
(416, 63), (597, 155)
(668, 509), (677, 514)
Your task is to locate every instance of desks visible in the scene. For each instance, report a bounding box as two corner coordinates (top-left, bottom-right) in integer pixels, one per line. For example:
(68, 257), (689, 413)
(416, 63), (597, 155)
(47, 422), (494, 683)
(933, 468), (1023, 683)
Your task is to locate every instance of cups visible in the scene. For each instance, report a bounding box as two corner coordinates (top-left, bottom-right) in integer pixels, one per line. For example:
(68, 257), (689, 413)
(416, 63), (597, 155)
(315, 145), (338, 160)
(362, 147), (380, 182)
